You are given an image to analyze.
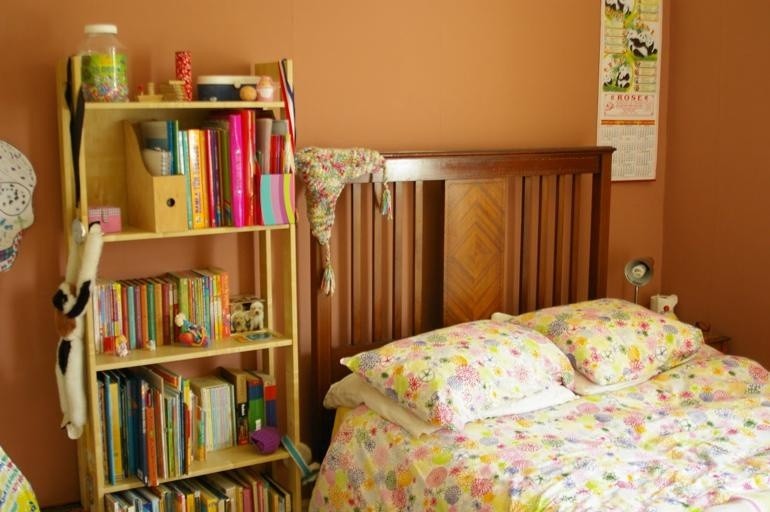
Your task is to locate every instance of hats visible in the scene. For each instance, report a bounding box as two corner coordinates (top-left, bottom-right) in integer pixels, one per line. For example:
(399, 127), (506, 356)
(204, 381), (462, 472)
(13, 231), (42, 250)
(295, 146), (385, 243)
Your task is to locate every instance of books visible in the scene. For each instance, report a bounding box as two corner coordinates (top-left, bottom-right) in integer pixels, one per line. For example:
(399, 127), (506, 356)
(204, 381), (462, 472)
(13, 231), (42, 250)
(96, 363), (294, 512)
(138, 108), (291, 227)
(90, 264), (231, 356)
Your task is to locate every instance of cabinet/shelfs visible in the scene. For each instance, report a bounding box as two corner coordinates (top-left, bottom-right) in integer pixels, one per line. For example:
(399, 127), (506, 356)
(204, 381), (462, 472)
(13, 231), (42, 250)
(55, 59), (318, 512)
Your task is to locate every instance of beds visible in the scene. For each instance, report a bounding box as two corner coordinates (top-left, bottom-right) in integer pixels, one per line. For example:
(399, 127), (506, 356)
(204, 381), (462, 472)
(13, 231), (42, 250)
(303, 146), (769, 512)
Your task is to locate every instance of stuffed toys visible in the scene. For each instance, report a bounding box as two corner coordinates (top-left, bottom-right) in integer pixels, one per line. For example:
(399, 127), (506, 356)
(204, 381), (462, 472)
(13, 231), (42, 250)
(50, 216), (106, 439)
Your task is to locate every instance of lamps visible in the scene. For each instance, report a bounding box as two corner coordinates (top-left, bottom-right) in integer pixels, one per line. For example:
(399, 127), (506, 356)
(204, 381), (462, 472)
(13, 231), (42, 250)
(624, 257), (655, 305)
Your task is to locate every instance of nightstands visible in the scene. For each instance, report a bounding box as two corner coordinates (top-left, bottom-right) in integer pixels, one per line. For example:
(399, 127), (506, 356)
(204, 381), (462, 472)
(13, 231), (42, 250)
(703, 330), (728, 354)
